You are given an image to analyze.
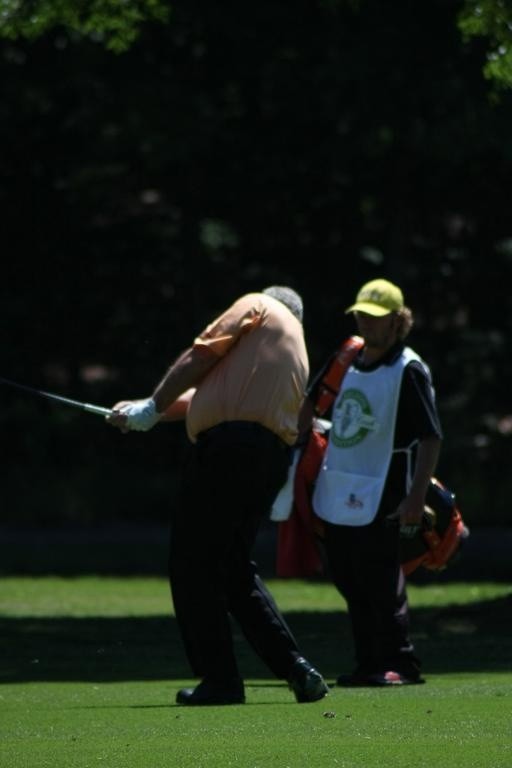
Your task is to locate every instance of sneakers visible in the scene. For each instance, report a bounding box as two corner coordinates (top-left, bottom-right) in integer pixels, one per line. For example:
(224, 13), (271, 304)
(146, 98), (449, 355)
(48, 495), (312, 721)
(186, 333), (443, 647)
(288, 657), (327, 702)
(370, 668), (408, 685)
(176, 687), (244, 704)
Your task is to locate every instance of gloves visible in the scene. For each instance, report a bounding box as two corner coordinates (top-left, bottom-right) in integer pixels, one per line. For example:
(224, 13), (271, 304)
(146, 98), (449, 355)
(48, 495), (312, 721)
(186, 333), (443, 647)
(126, 398), (163, 433)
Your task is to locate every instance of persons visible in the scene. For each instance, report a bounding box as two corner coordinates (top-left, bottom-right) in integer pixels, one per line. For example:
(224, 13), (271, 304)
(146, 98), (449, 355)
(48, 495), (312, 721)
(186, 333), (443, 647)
(104, 286), (327, 706)
(300, 277), (443, 687)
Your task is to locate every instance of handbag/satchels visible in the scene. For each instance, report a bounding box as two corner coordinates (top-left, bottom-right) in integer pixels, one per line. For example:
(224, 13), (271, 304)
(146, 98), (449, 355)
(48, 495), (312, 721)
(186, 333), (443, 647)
(278, 421), (470, 578)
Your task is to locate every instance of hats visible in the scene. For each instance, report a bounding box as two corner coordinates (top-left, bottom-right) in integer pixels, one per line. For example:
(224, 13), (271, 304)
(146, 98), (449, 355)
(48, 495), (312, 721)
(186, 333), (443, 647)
(346, 279), (403, 317)
